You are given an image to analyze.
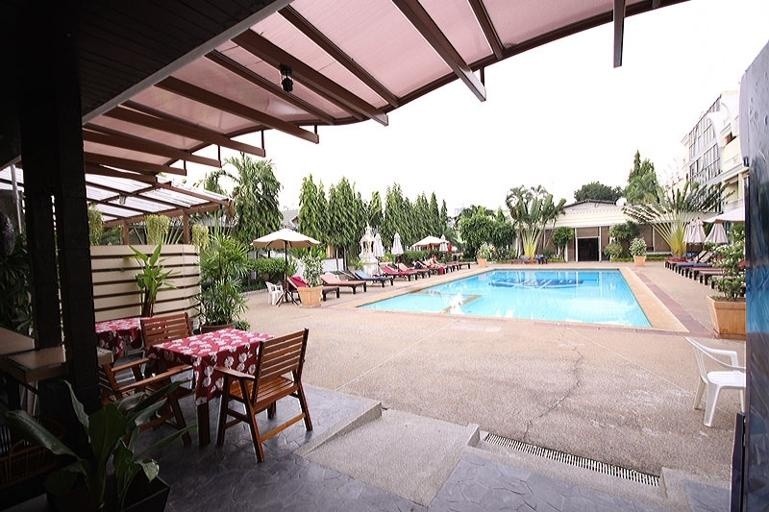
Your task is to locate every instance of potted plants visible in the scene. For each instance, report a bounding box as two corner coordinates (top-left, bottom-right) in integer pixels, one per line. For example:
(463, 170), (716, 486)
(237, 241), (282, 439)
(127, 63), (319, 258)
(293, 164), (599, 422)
(477, 242), (497, 268)
(193, 222), (248, 334)
(706, 213), (748, 343)
(627, 236), (650, 265)
(1, 373), (201, 512)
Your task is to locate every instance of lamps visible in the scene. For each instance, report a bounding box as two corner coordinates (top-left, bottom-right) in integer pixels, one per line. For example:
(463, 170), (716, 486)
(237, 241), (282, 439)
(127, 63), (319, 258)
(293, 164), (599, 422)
(280, 58), (296, 95)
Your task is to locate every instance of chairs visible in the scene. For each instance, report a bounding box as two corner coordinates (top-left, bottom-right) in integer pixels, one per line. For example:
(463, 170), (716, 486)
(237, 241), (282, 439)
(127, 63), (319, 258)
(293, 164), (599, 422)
(661, 243), (747, 292)
(684, 333), (748, 429)
(91, 347), (195, 457)
(131, 306), (202, 417)
(265, 231), (477, 311)
(212, 322), (310, 462)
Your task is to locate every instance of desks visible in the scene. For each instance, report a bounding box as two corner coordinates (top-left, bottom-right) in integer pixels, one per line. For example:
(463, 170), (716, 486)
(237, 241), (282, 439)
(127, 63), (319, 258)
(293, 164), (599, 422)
(0, 325), (41, 362)
(93, 311), (160, 365)
(3, 341), (115, 438)
(143, 325), (283, 445)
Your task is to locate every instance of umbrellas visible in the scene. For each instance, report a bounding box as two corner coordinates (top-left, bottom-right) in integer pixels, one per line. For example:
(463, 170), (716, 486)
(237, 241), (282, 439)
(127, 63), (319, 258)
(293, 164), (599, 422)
(439, 234), (449, 257)
(391, 231), (404, 263)
(411, 235), (450, 250)
(373, 233), (385, 265)
(681, 219), (729, 262)
(249, 229), (322, 301)
